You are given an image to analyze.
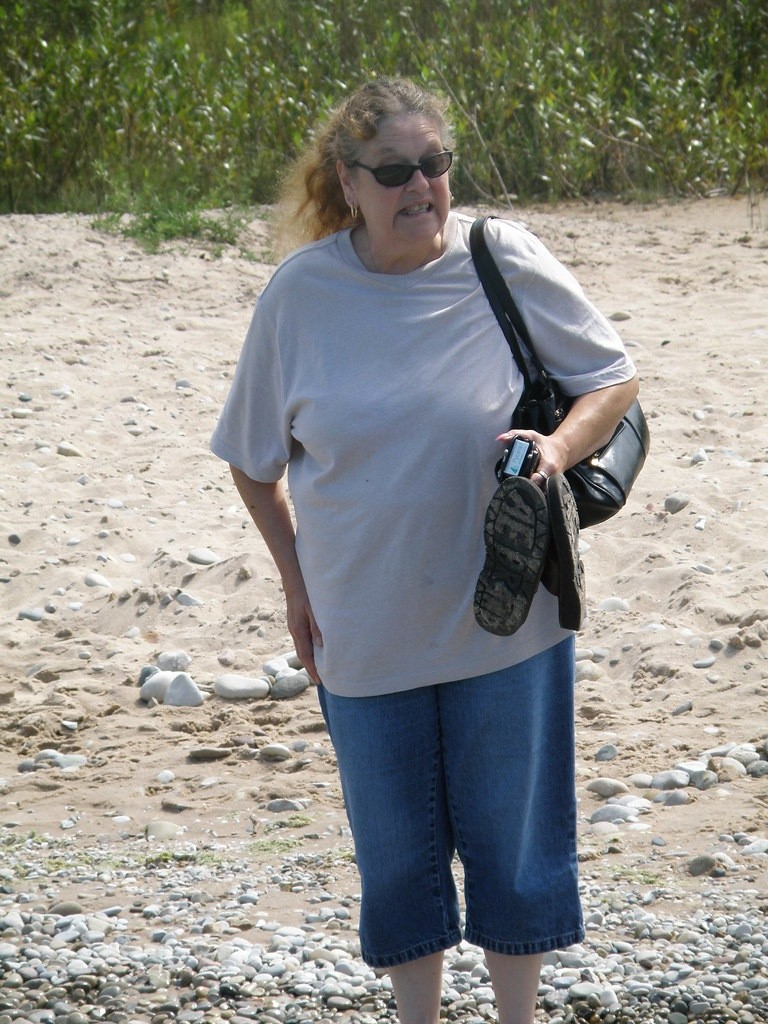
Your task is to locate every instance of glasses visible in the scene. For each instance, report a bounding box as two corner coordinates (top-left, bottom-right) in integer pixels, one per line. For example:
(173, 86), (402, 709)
(353, 147), (453, 187)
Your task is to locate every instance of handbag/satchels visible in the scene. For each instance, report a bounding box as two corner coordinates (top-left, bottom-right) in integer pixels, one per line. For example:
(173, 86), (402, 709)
(469, 215), (650, 529)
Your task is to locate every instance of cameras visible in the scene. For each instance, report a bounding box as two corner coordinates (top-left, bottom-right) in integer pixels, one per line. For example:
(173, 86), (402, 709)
(499, 434), (541, 478)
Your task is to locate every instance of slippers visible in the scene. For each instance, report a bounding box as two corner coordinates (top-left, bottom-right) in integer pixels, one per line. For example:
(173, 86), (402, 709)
(536, 470), (586, 631)
(473, 476), (551, 638)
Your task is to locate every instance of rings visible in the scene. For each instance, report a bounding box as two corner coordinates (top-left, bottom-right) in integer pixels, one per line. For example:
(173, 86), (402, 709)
(536, 470), (549, 480)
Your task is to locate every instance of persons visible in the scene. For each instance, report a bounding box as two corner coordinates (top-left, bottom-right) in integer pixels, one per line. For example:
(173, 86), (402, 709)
(208, 80), (639, 1024)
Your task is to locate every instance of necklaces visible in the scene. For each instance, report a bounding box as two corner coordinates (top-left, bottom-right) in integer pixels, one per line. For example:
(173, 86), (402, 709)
(368, 226), (445, 274)
